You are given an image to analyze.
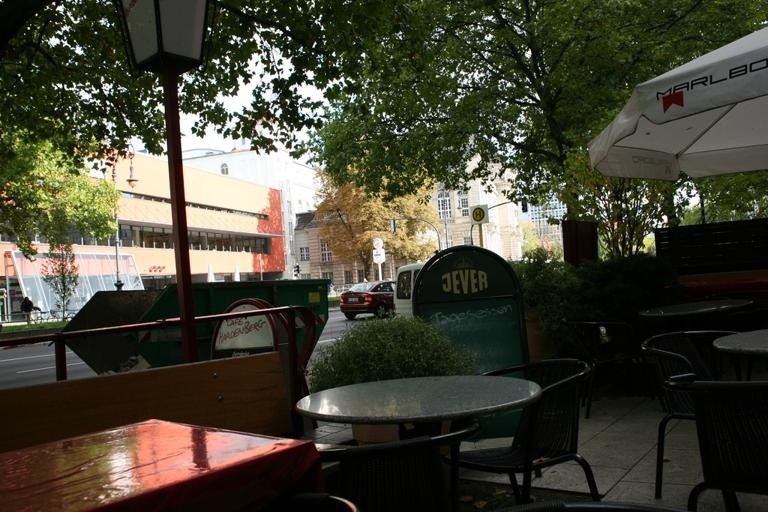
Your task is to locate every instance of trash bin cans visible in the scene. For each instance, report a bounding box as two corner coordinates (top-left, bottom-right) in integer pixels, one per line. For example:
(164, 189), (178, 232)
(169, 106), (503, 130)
(62, 279), (331, 372)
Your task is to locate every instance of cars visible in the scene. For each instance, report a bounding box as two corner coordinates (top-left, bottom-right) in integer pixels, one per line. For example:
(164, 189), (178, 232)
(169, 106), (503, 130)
(338, 280), (396, 320)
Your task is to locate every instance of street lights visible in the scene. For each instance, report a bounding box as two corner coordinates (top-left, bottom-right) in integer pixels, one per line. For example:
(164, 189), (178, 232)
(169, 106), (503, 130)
(114, 0), (219, 361)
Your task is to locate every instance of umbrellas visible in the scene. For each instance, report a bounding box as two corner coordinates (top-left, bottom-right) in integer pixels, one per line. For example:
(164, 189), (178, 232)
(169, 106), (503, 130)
(587, 25), (768, 181)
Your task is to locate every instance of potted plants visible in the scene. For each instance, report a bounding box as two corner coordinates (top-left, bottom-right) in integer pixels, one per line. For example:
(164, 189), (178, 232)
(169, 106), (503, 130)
(306, 317), (472, 440)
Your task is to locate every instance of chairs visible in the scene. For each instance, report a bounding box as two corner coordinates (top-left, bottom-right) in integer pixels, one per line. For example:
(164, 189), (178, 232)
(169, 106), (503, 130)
(274, 421), (478, 512)
(538, 316), (768, 511)
(444, 358), (600, 512)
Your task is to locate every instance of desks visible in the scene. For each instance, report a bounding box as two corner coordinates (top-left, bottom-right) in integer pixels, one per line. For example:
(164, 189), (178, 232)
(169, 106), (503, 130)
(1, 417), (321, 510)
(296, 376), (544, 439)
(636, 269), (768, 376)
